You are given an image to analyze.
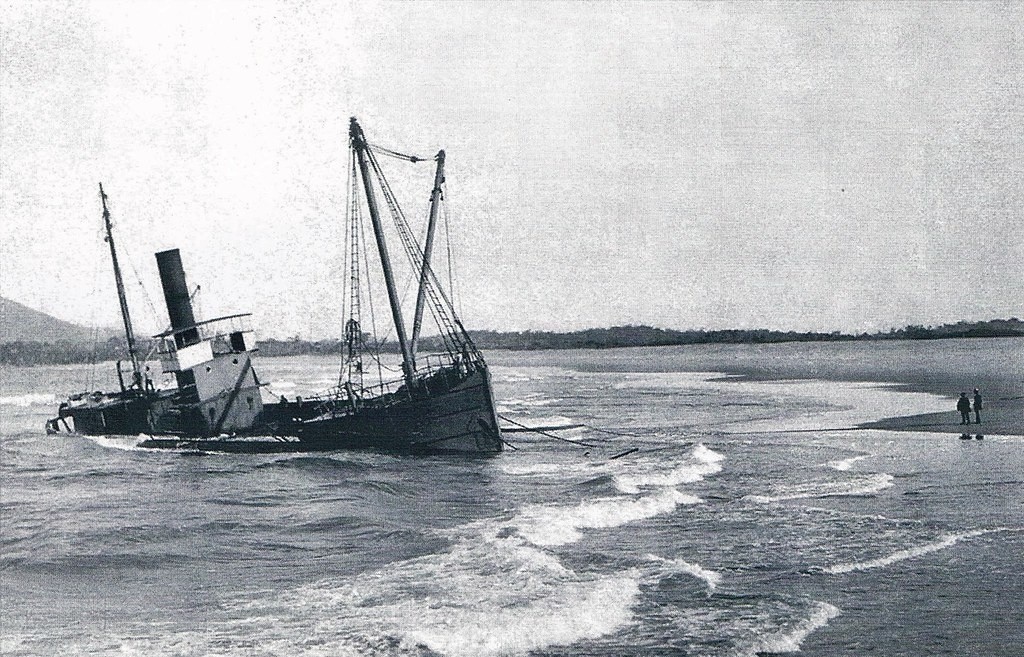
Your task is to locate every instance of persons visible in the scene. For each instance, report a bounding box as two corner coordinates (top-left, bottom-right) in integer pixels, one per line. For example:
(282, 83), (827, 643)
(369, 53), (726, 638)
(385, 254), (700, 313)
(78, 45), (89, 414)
(974, 389), (983, 424)
(957, 392), (970, 425)
(144, 366), (155, 394)
(130, 366), (143, 392)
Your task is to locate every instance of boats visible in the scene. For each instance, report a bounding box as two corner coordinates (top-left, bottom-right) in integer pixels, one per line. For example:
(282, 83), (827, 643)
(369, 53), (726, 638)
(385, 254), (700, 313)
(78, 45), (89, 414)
(44, 115), (509, 453)
